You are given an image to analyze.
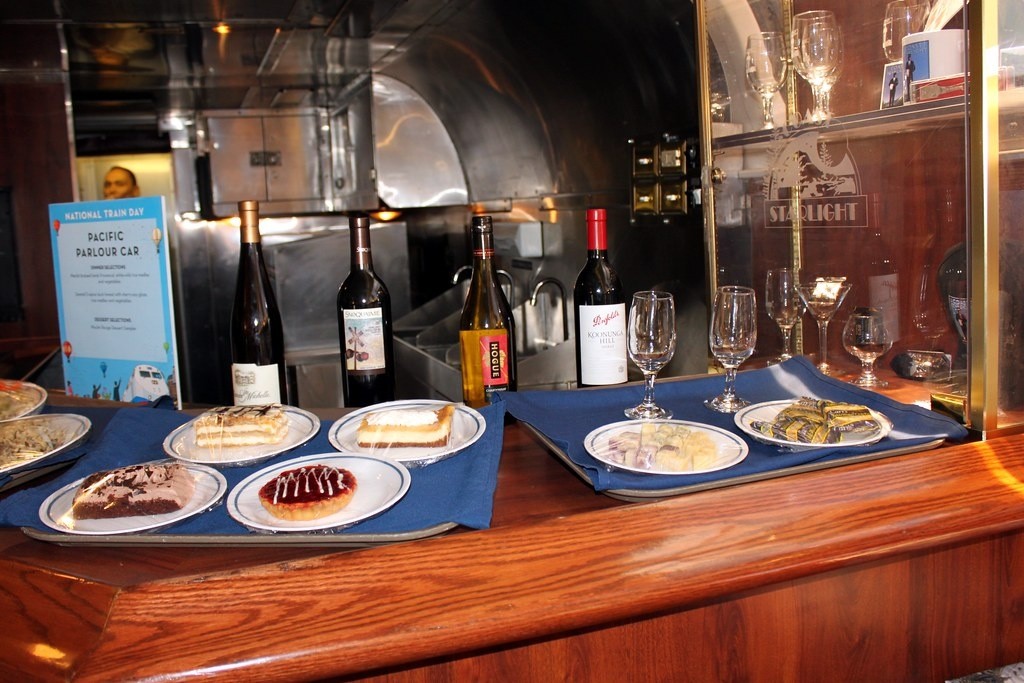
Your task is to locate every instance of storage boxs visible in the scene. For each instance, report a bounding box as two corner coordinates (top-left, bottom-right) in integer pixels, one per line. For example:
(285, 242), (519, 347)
(910, 65), (1014, 104)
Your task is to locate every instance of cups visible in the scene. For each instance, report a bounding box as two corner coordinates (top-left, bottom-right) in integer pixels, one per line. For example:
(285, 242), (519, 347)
(998, 66), (1016, 91)
(882, 0), (931, 62)
(901, 30), (969, 106)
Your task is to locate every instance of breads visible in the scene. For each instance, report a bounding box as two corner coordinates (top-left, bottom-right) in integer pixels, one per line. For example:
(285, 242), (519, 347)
(356, 405), (455, 448)
(72, 464), (195, 520)
(608, 422), (718, 471)
(769, 397), (877, 445)
(191, 404), (288, 447)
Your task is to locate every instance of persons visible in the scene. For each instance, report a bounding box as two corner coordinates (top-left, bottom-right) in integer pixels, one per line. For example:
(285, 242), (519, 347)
(889, 72), (898, 106)
(906, 54), (915, 98)
(104, 166), (139, 199)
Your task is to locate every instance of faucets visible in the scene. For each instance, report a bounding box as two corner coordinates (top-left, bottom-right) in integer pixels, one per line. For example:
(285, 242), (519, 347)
(531, 277), (569, 342)
(450, 265), (473, 286)
(496, 269), (515, 310)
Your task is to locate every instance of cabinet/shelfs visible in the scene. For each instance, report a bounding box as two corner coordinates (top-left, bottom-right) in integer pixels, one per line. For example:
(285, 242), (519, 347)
(198, 108), (335, 219)
(692, 0), (1024, 443)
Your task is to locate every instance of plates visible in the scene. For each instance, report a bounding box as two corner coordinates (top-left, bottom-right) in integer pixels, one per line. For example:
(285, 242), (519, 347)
(39, 459), (227, 535)
(584, 419), (749, 475)
(0, 381), (48, 422)
(0, 414), (91, 473)
(163, 405), (321, 467)
(734, 400), (893, 448)
(327, 399), (486, 462)
(227, 452), (411, 531)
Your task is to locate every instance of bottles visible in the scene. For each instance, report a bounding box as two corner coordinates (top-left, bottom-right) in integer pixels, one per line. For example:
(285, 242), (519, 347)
(937, 246), (966, 369)
(231, 201), (288, 405)
(460, 216), (518, 409)
(574, 208), (628, 388)
(867, 229), (900, 348)
(336, 218), (395, 408)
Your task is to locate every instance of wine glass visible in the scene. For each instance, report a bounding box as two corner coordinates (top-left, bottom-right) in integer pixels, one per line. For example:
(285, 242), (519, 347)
(624, 290), (676, 420)
(704, 286), (757, 414)
(842, 311), (894, 386)
(792, 279), (854, 377)
(765, 266), (807, 366)
(791, 10), (844, 123)
(745, 31), (787, 133)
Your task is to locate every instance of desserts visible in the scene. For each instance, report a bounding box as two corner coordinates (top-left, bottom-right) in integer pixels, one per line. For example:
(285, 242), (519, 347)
(259, 465), (357, 521)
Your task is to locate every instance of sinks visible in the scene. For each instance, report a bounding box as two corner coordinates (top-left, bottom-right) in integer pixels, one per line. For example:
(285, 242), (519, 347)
(393, 323), (552, 403)
